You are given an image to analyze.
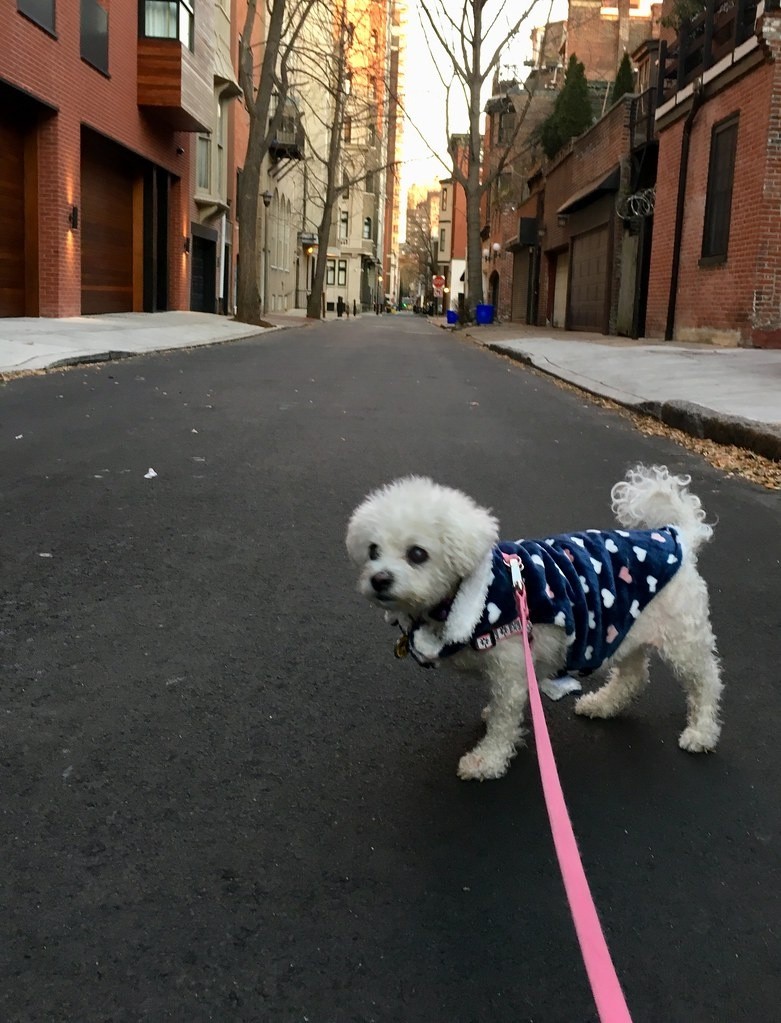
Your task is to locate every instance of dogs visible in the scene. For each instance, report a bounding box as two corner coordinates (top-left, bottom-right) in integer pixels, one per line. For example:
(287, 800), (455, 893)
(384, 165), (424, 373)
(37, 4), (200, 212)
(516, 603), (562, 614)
(343, 461), (723, 785)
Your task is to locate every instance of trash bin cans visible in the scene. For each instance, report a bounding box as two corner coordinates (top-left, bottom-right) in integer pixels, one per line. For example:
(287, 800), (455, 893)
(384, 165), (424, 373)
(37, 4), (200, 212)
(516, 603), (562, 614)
(476, 305), (494, 326)
(447, 310), (458, 323)
